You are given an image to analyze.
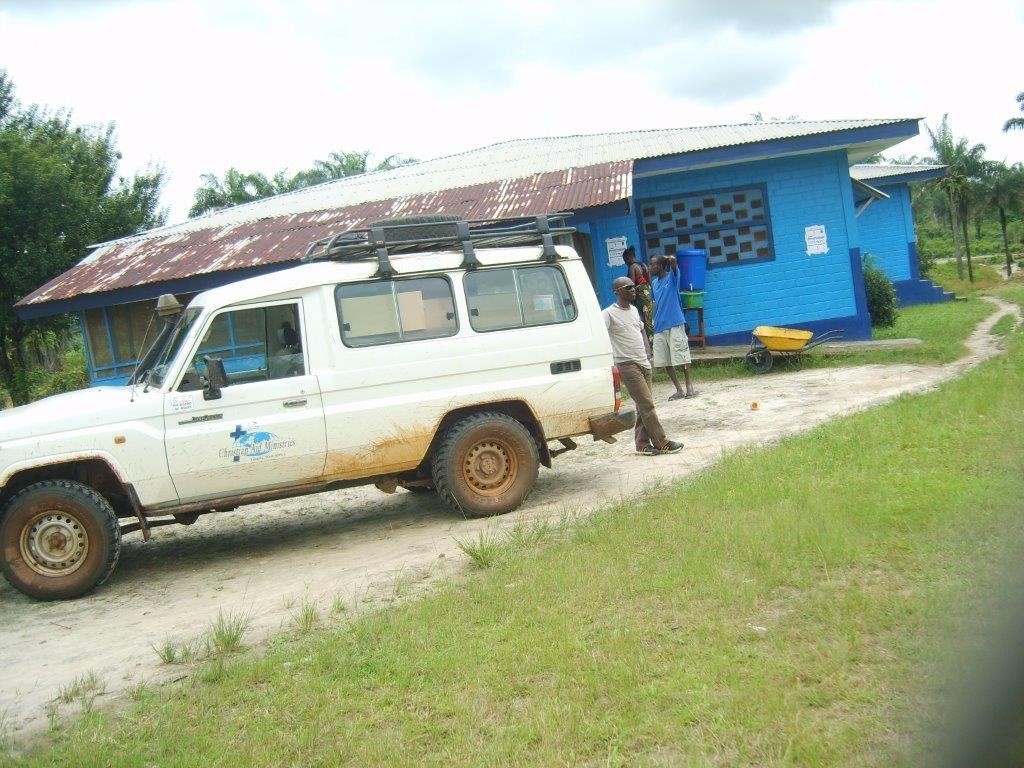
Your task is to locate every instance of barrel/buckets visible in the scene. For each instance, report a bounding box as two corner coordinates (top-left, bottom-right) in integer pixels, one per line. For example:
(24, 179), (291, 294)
(677, 248), (708, 290)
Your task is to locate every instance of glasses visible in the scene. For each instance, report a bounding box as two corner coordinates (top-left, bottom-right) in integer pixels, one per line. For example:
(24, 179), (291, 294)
(617, 283), (637, 292)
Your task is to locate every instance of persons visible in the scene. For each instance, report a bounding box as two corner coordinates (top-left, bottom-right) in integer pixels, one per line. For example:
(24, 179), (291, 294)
(649, 255), (699, 401)
(601, 277), (684, 456)
(623, 246), (653, 355)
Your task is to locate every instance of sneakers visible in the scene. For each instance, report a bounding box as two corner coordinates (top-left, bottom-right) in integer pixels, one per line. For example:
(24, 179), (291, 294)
(652, 441), (684, 455)
(634, 445), (659, 455)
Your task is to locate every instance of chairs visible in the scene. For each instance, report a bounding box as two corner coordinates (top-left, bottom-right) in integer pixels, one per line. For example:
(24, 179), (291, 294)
(276, 327), (301, 379)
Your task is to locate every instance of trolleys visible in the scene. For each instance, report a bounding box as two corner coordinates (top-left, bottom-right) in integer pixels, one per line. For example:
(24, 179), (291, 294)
(744, 325), (846, 373)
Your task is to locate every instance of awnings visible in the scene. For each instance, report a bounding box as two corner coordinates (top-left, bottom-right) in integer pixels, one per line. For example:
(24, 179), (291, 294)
(13, 159), (636, 307)
(850, 176), (890, 218)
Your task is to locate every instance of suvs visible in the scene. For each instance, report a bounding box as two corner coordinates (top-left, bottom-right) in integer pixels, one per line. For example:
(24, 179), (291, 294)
(0, 212), (635, 600)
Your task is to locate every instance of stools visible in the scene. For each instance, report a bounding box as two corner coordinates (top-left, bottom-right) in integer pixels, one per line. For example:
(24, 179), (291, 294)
(683, 307), (707, 353)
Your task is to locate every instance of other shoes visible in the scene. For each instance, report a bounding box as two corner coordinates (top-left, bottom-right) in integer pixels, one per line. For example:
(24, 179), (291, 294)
(683, 391), (703, 399)
(668, 392), (686, 401)
